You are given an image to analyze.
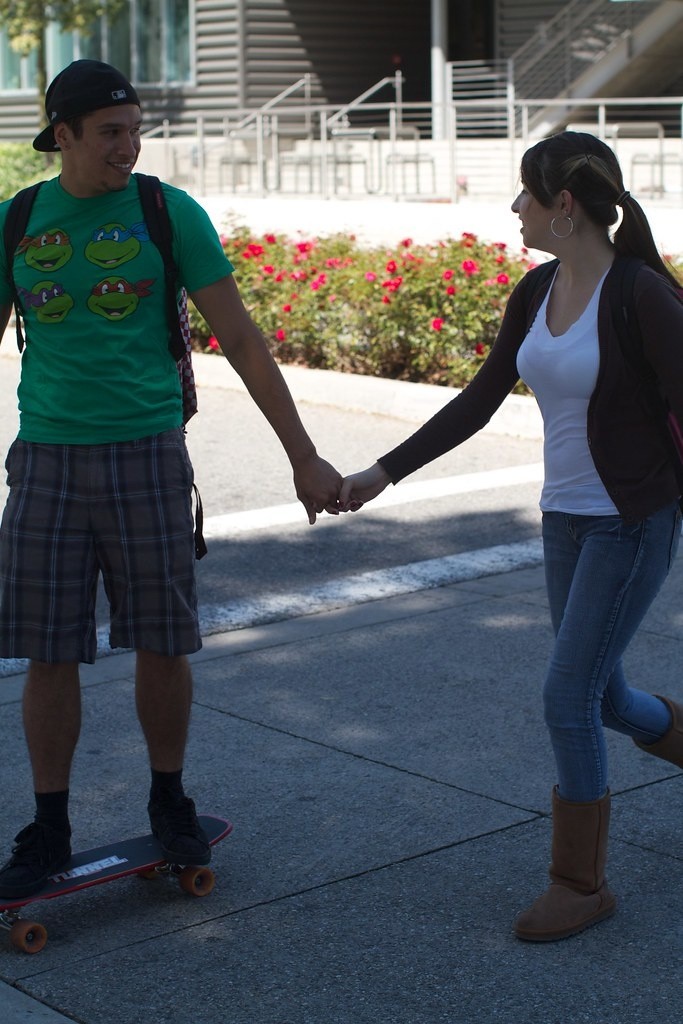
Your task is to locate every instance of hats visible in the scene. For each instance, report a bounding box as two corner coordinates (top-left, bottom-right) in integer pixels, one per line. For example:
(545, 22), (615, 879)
(32, 61), (140, 152)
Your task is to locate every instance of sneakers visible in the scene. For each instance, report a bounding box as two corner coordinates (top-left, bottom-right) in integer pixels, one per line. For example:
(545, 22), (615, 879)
(147, 796), (212, 864)
(0, 822), (72, 900)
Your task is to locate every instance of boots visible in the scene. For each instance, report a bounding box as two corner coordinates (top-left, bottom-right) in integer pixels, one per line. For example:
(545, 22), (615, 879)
(516, 785), (616, 942)
(633, 693), (683, 768)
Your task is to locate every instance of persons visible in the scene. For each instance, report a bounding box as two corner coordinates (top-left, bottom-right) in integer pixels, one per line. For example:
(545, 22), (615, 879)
(0, 57), (344, 871)
(352, 131), (682, 943)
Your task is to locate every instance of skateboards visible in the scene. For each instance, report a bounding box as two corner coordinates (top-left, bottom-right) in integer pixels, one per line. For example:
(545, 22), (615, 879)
(0, 812), (234, 955)
